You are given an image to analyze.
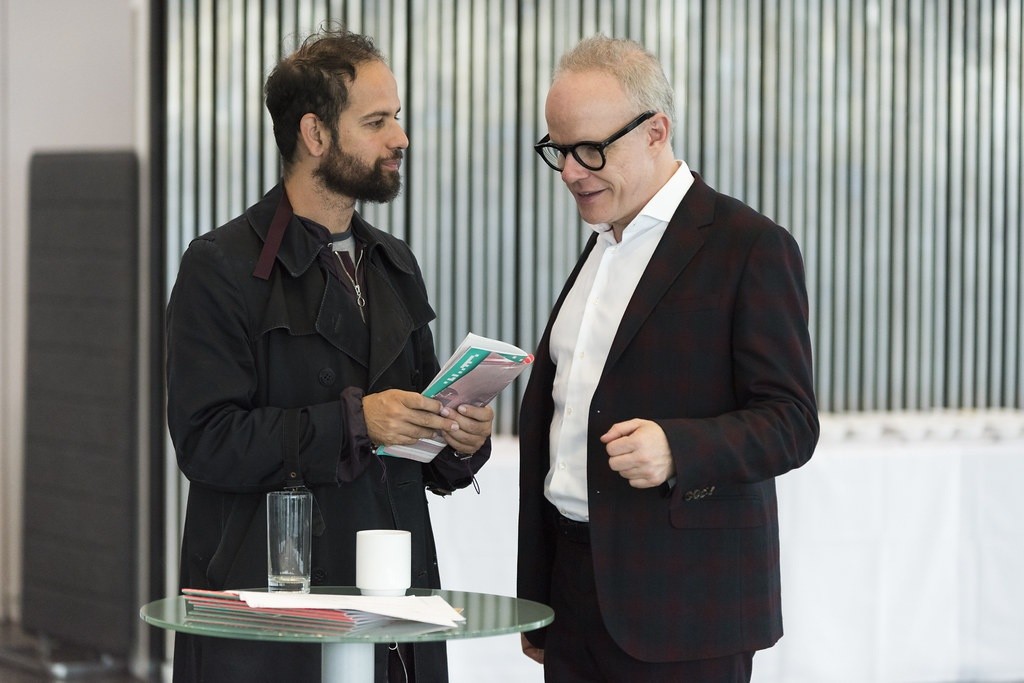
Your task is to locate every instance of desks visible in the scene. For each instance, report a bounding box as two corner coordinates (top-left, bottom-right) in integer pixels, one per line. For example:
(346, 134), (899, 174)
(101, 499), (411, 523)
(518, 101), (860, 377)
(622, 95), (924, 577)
(140, 587), (555, 683)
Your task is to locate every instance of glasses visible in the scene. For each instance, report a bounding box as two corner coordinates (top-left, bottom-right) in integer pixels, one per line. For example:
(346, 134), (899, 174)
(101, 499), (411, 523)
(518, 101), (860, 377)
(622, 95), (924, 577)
(532, 108), (659, 170)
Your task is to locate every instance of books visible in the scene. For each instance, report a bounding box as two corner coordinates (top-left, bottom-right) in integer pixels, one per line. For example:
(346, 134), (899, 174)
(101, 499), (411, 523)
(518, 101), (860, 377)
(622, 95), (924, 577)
(182, 588), (403, 636)
(372, 332), (534, 463)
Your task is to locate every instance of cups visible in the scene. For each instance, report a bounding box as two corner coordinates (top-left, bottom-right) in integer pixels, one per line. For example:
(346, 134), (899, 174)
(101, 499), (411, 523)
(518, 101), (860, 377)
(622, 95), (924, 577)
(267, 492), (312, 594)
(356, 530), (412, 596)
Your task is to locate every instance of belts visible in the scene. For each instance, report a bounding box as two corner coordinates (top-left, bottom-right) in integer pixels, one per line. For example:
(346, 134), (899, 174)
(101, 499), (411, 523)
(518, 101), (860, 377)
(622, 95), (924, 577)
(550, 515), (589, 545)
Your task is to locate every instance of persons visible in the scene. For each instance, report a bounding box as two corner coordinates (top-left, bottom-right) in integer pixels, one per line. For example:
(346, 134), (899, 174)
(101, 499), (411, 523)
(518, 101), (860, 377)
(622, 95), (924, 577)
(165, 30), (494, 683)
(516, 35), (819, 683)
